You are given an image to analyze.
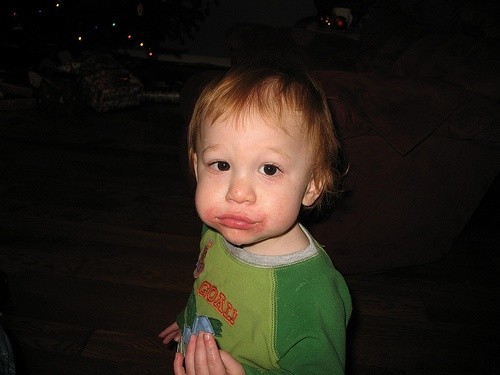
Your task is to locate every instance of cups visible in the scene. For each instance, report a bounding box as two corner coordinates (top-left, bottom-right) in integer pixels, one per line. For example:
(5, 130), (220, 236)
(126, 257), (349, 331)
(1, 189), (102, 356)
(333, 8), (353, 30)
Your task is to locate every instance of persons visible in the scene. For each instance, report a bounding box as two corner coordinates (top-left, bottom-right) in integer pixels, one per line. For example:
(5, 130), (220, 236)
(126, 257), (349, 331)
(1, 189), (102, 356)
(158, 49), (352, 375)
